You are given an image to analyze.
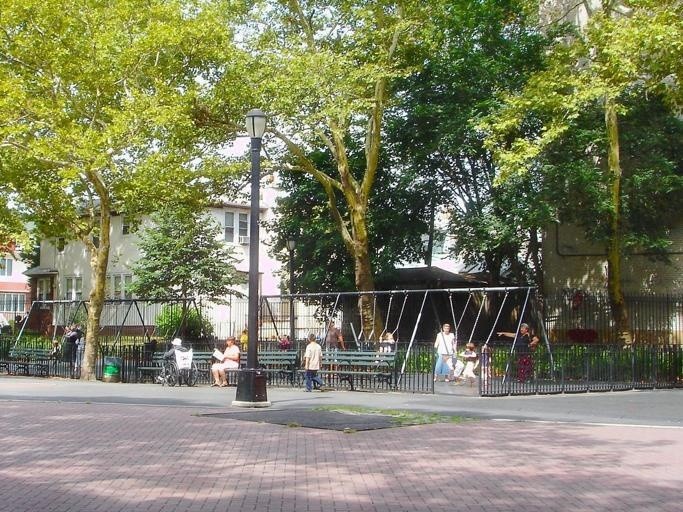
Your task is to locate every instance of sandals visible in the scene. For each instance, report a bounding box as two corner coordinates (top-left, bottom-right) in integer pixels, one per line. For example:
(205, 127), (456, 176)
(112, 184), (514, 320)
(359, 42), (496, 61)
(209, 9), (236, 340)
(220, 383), (229, 387)
(210, 383), (219, 387)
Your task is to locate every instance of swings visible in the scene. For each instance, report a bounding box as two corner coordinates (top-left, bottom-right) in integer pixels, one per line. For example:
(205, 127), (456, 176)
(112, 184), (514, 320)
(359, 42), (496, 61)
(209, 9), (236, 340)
(463, 291), (510, 362)
(277, 297), (297, 351)
(379, 294), (408, 352)
(449, 292), (473, 370)
(358, 294), (377, 352)
(40, 301), (179, 358)
(305, 296), (324, 345)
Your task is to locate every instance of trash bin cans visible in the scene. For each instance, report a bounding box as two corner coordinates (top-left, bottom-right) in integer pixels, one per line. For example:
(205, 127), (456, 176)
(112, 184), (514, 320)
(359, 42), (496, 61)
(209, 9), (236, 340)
(102, 356), (122, 383)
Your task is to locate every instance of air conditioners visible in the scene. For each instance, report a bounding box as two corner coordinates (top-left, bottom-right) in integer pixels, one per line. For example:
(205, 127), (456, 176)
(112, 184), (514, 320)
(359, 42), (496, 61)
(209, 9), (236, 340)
(239, 236), (250, 245)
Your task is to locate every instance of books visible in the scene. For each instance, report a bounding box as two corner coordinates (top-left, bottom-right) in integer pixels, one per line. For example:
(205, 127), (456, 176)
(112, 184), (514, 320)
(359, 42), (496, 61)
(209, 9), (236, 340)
(212, 347), (224, 361)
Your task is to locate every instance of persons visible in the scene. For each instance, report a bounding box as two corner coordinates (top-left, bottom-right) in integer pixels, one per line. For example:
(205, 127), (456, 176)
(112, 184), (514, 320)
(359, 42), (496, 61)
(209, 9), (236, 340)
(302, 334), (326, 393)
(432, 323), (457, 382)
(476, 344), (492, 385)
(456, 342), (478, 383)
(496, 322), (540, 383)
(240, 328), (249, 352)
(323, 319), (346, 379)
(377, 328), (394, 362)
(314, 331), (323, 346)
(210, 336), (240, 387)
(156, 336), (187, 381)
(48, 322), (87, 365)
(277, 334), (289, 352)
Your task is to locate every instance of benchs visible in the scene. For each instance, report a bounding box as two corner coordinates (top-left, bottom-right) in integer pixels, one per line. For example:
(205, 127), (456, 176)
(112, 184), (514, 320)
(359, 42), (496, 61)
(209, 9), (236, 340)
(0, 346), (59, 379)
(134, 347), (398, 392)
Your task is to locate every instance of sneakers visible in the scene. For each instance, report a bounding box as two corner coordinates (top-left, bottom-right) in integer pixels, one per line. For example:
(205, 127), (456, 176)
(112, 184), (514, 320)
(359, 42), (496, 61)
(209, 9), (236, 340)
(157, 376), (163, 381)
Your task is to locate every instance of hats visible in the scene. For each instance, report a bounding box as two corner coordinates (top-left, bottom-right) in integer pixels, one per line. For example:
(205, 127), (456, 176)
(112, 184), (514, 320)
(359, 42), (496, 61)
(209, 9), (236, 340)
(171, 338), (182, 345)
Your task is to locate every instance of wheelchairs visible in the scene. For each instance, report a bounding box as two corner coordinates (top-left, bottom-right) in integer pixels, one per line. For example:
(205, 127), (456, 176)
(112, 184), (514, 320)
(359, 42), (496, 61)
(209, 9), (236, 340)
(161, 356), (199, 387)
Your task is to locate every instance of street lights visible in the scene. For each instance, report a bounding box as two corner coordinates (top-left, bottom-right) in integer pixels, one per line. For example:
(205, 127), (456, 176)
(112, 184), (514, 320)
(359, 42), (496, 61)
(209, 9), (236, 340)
(234, 108), (271, 404)
(284, 233), (298, 351)
